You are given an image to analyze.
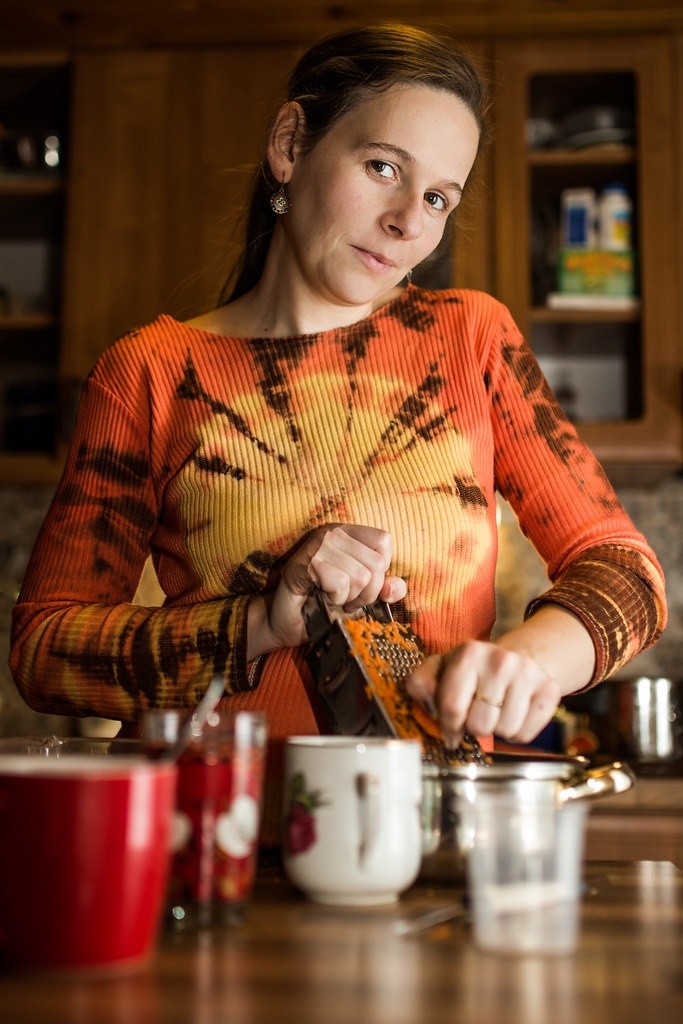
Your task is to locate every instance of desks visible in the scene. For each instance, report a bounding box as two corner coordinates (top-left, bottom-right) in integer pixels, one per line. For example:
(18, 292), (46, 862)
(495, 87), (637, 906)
(1, 831), (683, 1024)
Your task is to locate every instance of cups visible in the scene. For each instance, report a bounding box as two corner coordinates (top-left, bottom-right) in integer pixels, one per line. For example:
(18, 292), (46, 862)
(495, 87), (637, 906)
(144, 709), (266, 931)
(285, 736), (423, 906)
(0, 755), (178, 983)
(465, 781), (586, 958)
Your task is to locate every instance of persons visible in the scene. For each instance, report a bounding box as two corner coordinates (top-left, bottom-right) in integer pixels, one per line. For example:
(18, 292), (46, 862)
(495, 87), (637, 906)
(6, 13), (668, 771)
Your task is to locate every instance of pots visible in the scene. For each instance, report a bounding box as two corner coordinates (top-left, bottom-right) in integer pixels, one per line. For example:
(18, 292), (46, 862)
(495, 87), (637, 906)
(421, 751), (638, 892)
(582, 678), (673, 762)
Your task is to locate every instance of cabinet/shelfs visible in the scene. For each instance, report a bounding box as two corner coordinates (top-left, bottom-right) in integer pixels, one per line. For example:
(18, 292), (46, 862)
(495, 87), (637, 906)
(0, 0), (683, 500)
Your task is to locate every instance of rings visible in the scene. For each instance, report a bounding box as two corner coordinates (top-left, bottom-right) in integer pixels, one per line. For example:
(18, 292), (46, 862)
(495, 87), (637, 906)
(475, 691), (505, 707)
